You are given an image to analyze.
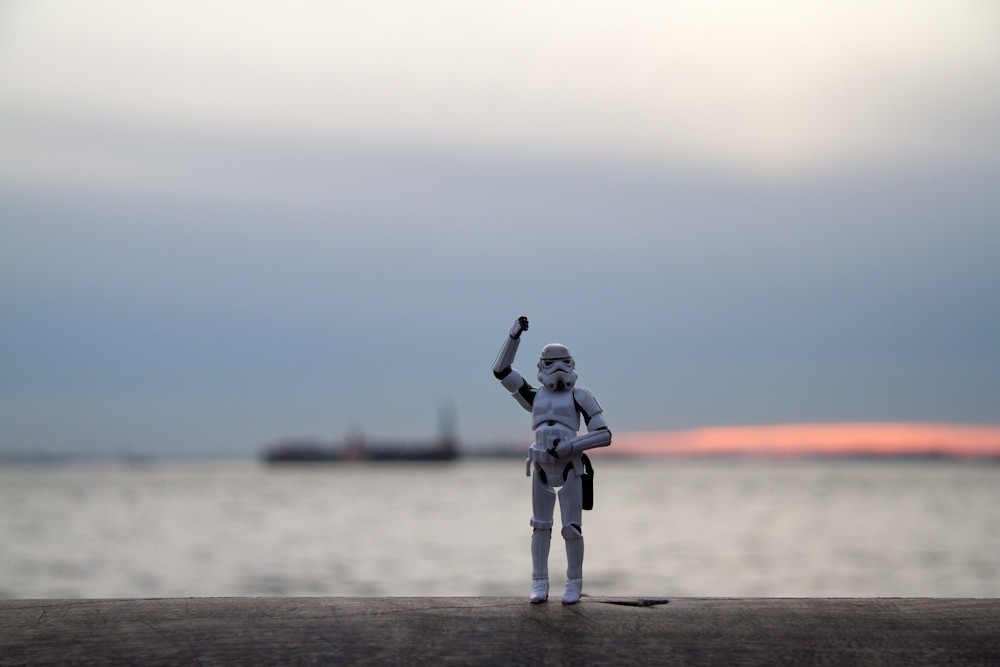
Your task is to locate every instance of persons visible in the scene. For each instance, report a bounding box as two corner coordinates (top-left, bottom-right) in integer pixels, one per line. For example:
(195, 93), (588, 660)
(493, 315), (612, 604)
